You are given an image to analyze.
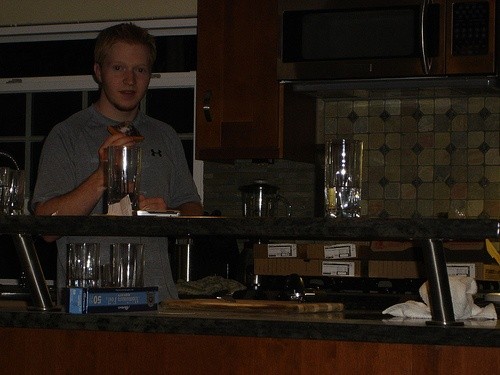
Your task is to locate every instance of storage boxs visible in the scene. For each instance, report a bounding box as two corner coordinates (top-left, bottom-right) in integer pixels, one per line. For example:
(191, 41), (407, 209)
(68, 287), (157, 314)
(252, 242), (500, 282)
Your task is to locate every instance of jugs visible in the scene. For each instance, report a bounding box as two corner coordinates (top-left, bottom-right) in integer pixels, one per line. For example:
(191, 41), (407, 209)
(236, 179), (292, 217)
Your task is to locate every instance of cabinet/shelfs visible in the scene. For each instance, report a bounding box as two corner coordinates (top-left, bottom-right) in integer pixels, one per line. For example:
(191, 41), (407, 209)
(195, 0), (314, 161)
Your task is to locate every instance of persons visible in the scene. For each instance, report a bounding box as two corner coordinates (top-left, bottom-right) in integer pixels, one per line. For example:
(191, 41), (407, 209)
(29, 23), (204, 300)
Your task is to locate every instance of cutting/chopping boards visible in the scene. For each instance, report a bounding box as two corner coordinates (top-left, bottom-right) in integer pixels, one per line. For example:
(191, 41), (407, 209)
(161, 296), (345, 314)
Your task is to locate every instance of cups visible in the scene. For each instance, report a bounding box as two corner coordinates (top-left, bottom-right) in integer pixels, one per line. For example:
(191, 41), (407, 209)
(102, 242), (145, 289)
(323, 138), (365, 218)
(66, 242), (99, 287)
(1, 168), (27, 215)
(107, 145), (142, 215)
